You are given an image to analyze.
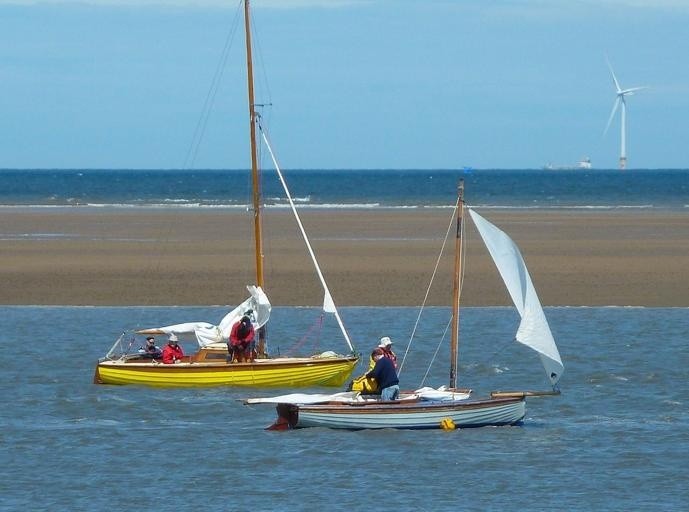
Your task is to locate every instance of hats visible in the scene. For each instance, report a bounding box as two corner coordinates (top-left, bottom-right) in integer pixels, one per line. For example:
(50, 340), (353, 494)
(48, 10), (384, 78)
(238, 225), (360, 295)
(147, 336), (154, 340)
(378, 337), (394, 348)
(168, 336), (180, 342)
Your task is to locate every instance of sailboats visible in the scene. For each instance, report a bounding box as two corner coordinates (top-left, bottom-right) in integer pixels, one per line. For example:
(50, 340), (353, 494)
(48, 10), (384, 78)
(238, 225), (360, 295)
(276, 177), (526, 429)
(94, 0), (360, 389)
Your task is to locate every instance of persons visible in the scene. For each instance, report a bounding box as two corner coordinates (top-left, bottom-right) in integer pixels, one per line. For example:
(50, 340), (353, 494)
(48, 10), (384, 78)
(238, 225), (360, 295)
(163, 335), (184, 364)
(378, 337), (397, 369)
(227, 316), (255, 364)
(139, 337), (163, 359)
(366, 348), (400, 402)
